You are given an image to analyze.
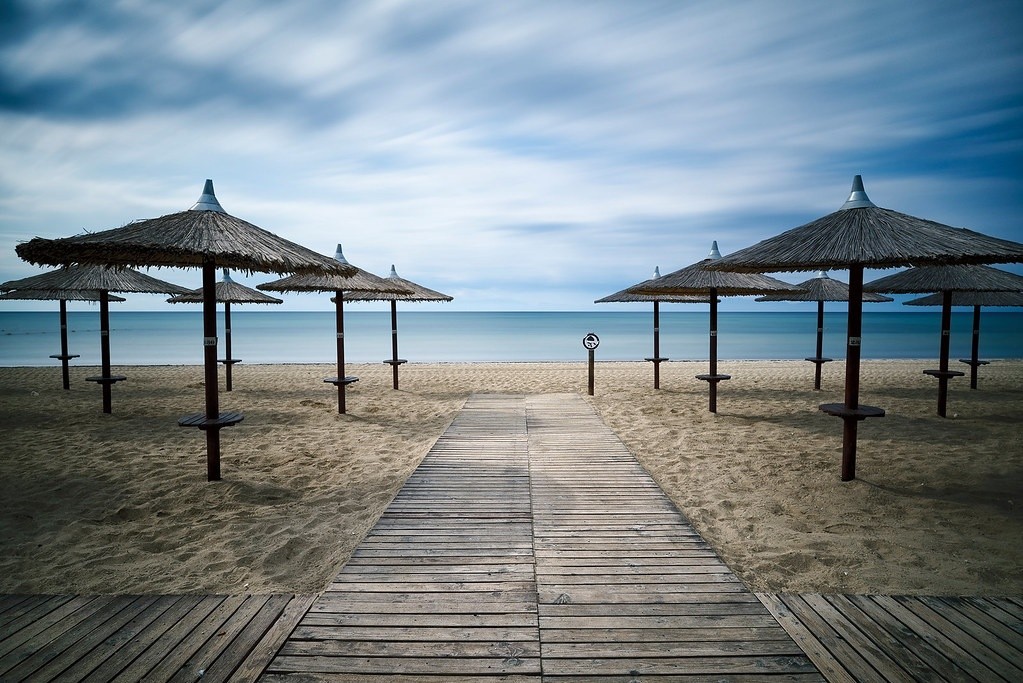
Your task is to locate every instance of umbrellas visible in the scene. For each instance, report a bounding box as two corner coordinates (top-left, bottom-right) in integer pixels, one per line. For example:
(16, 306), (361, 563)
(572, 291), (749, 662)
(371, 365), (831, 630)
(0, 179), (456, 483)
(594, 175), (1023, 480)
